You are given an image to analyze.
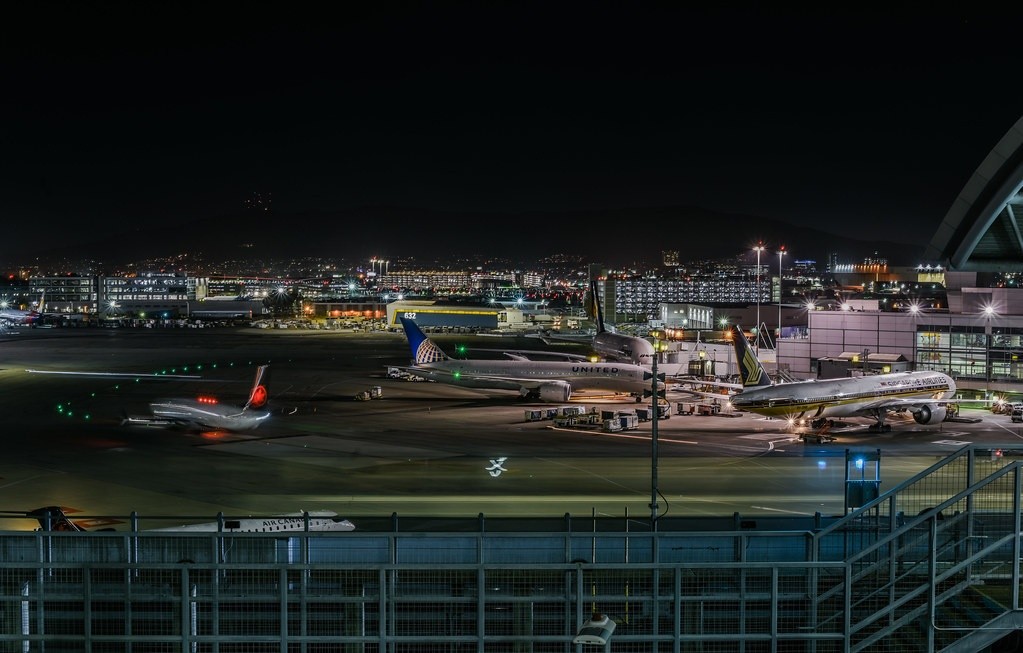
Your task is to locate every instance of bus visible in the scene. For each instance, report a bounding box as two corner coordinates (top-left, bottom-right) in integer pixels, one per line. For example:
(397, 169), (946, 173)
(192, 311), (254, 319)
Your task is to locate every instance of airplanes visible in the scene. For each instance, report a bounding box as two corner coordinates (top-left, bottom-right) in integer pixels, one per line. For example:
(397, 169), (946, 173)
(674, 322), (996, 445)
(26, 505), (357, 536)
(384, 281), (665, 401)
(120, 363), (275, 440)
(0, 292), (50, 326)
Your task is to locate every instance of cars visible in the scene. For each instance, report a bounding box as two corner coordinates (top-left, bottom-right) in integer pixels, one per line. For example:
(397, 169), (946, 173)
(52, 317), (481, 334)
(386, 367), (434, 382)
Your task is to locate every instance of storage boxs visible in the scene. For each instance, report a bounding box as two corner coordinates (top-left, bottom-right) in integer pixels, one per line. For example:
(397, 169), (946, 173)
(388, 366), (399, 375)
(526, 402), (721, 431)
(142, 319), (330, 329)
(361, 385), (382, 399)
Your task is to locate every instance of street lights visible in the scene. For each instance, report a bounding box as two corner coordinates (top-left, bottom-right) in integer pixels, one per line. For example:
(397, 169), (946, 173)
(776, 245), (789, 340)
(752, 241), (765, 361)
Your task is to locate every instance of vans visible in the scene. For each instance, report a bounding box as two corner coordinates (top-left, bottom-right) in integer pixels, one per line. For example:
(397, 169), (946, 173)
(555, 318), (578, 330)
(992, 401), (1023, 423)
